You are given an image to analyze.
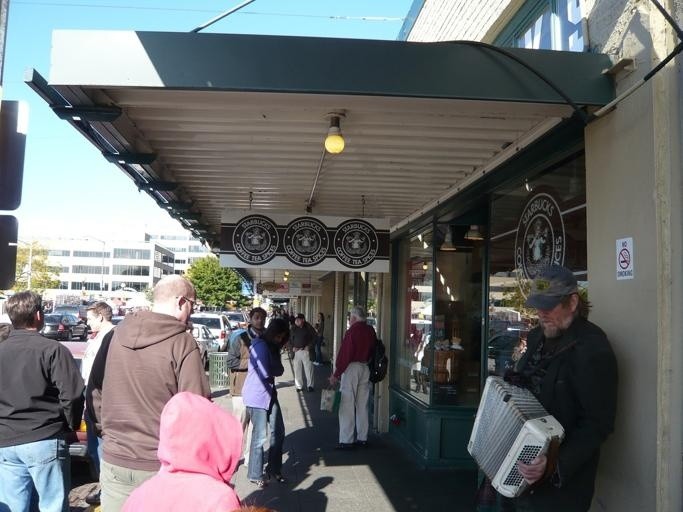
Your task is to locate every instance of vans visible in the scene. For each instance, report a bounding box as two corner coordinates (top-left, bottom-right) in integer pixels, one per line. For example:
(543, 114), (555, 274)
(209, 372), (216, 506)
(411, 319), (432, 346)
(53, 304), (91, 330)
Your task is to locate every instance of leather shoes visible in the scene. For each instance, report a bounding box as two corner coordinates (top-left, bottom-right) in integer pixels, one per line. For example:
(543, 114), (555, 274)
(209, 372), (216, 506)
(354, 439), (367, 448)
(297, 389), (304, 394)
(331, 440), (354, 450)
(306, 386), (314, 392)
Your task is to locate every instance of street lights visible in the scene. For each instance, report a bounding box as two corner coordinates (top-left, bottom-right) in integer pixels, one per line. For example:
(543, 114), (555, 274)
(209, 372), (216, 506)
(9, 240), (48, 290)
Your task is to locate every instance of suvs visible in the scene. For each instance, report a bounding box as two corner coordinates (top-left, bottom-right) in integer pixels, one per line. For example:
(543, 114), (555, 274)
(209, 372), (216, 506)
(190, 313), (233, 352)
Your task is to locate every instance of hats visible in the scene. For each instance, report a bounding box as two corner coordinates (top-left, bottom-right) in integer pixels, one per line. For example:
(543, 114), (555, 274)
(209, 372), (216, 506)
(522, 265), (577, 311)
(293, 313), (304, 319)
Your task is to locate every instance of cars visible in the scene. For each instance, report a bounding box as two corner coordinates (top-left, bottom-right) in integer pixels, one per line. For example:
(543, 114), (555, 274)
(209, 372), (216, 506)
(58, 342), (91, 456)
(365, 317), (377, 332)
(488, 319), (533, 358)
(219, 311), (250, 328)
(39, 314), (88, 341)
(187, 323), (220, 369)
(110, 316), (125, 326)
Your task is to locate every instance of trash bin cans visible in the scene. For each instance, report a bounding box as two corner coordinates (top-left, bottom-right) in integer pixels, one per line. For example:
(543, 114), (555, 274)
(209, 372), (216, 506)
(208, 352), (229, 386)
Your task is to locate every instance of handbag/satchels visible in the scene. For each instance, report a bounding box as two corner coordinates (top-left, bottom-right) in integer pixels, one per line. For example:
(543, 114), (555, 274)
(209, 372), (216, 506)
(319, 382), (335, 412)
(320, 382), (340, 416)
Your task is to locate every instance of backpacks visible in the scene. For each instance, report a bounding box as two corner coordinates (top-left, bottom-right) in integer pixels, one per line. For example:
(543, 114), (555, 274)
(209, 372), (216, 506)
(366, 339), (387, 384)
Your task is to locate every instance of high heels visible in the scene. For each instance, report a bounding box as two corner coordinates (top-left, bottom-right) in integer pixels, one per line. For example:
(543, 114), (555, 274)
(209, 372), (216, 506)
(265, 463), (287, 483)
(251, 480), (268, 488)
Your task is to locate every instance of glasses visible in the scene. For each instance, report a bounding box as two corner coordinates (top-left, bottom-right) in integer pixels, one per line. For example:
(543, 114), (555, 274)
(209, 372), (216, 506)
(175, 296), (196, 310)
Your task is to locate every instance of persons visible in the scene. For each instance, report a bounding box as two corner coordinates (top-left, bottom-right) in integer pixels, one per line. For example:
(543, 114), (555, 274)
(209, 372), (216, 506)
(270, 308), (324, 391)
(0, 290), (85, 512)
(122, 391), (243, 512)
(329, 306), (377, 451)
(499, 264), (618, 511)
(227, 307), (288, 487)
(80, 301), (115, 481)
(85, 275), (211, 512)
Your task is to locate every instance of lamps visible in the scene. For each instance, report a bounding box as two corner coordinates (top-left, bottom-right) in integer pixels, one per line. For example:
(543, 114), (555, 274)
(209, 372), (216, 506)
(320, 108), (349, 157)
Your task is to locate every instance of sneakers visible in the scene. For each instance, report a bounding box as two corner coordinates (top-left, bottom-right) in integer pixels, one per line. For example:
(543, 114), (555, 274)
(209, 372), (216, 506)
(86, 489), (101, 504)
(312, 361), (319, 366)
